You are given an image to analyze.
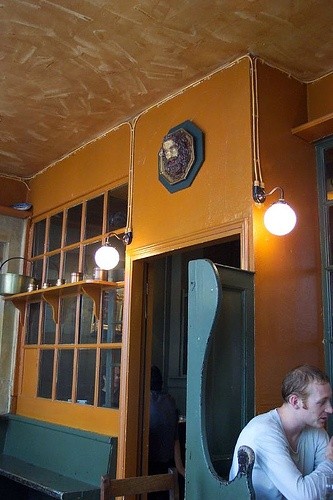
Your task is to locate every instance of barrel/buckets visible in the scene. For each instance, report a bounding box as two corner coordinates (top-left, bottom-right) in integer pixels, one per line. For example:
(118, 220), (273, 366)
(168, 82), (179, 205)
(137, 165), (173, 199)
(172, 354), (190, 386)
(0, 257), (37, 295)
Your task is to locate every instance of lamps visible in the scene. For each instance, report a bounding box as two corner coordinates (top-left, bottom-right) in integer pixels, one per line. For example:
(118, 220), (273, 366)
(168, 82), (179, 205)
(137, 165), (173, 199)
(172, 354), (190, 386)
(253, 186), (297, 237)
(95, 231), (133, 271)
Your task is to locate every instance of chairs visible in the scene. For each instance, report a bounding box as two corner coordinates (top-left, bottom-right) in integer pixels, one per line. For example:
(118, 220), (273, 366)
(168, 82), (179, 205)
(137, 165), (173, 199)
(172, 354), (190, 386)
(100, 467), (179, 500)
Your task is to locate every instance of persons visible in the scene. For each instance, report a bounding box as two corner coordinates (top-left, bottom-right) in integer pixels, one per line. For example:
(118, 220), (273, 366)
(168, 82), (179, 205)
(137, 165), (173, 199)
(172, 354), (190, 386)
(229, 365), (333, 500)
(147, 361), (185, 500)
(162, 133), (191, 180)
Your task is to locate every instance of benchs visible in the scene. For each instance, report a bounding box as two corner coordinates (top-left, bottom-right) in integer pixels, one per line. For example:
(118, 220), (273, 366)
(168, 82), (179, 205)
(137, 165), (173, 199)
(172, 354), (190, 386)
(185, 259), (255, 500)
(0, 413), (118, 500)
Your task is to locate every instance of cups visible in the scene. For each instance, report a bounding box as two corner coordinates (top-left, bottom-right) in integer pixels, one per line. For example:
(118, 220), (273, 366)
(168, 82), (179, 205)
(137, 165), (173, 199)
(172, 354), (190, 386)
(70, 272), (83, 283)
(93, 268), (108, 281)
(57, 279), (65, 286)
(43, 283), (52, 289)
